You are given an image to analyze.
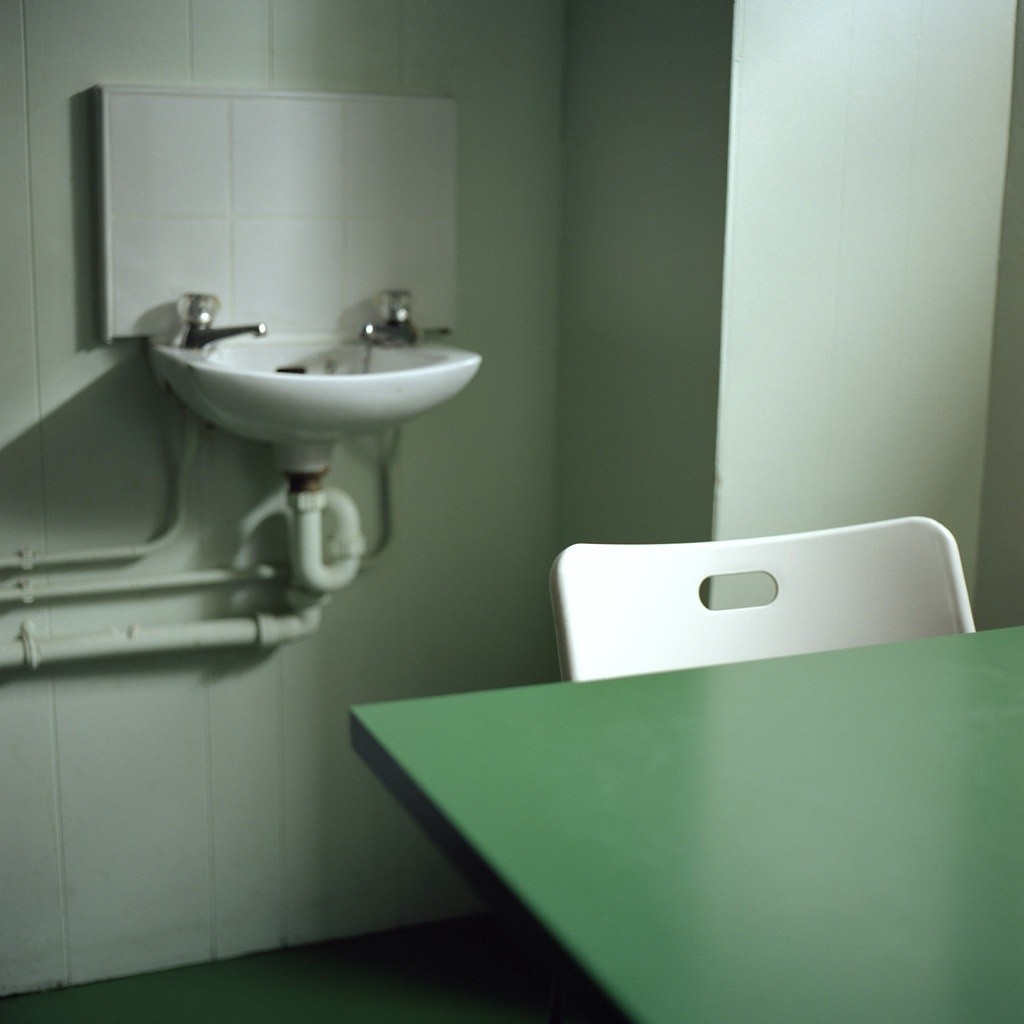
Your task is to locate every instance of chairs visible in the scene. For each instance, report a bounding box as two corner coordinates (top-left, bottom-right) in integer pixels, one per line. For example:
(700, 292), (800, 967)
(550, 515), (977, 683)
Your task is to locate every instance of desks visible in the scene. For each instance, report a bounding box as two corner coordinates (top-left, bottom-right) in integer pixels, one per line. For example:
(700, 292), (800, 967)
(349, 628), (1024, 1024)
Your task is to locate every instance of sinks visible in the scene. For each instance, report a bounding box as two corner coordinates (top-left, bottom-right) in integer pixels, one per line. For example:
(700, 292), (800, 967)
(147, 333), (482, 445)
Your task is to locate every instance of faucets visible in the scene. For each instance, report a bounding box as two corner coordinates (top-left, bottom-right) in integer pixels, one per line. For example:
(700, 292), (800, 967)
(178, 292), (268, 348)
(358, 288), (414, 344)
